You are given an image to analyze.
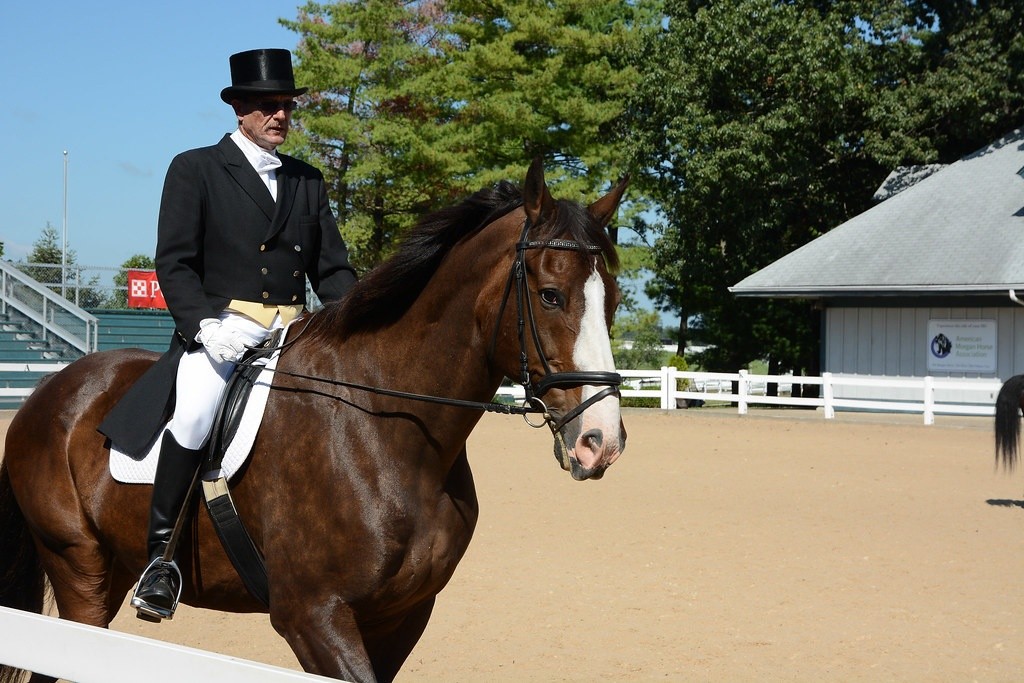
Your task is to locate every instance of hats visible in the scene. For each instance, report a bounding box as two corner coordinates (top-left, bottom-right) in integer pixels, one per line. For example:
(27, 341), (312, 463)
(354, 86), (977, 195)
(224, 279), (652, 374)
(220, 49), (309, 105)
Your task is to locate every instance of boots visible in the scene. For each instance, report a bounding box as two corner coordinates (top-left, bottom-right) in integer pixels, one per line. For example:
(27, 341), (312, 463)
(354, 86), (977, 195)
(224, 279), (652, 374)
(137, 428), (210, 619)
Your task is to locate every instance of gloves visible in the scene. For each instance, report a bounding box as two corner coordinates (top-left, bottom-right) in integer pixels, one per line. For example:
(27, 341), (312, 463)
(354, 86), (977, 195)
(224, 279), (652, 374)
(200, 318), (252, 363)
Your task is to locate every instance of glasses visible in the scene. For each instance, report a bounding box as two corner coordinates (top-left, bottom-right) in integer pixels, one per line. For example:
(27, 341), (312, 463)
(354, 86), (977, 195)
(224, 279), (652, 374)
(241, 99), (298, 112)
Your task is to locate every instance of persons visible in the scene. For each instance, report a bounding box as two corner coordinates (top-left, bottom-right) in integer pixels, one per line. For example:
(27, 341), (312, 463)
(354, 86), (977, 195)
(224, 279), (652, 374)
(96, 49), (359, 623)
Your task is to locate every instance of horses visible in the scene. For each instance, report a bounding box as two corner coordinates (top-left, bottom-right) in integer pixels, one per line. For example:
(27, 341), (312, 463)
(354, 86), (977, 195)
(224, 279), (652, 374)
(0, 149), (631, 683)
